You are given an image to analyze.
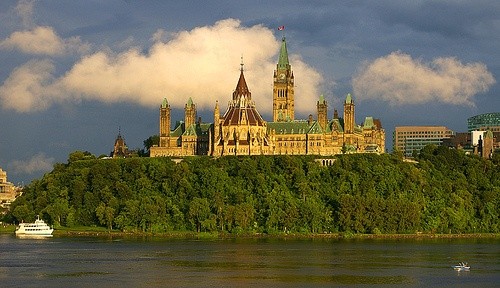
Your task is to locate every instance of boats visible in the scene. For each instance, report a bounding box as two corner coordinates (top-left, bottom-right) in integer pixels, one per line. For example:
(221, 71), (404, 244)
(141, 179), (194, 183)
(17, 235), (53, 242)
(15, 215), (54, 236)
